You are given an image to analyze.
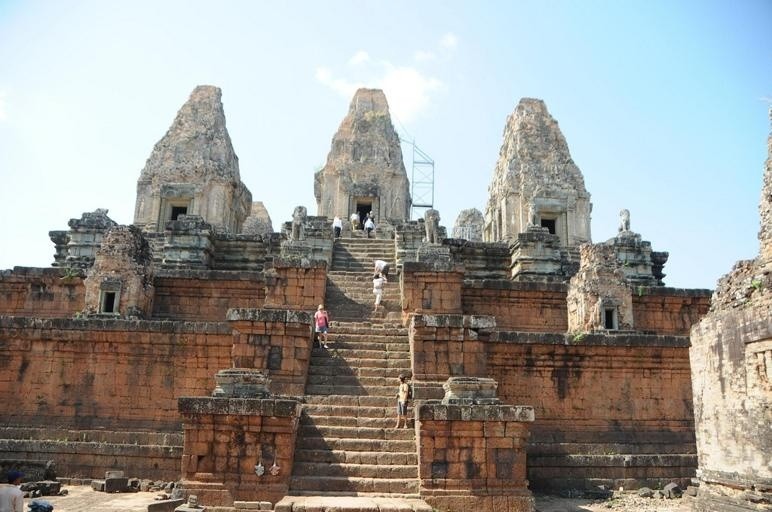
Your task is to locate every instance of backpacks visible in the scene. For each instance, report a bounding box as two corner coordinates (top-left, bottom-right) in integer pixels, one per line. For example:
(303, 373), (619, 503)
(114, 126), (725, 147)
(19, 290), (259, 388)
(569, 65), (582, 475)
(408, 384), (412, 399)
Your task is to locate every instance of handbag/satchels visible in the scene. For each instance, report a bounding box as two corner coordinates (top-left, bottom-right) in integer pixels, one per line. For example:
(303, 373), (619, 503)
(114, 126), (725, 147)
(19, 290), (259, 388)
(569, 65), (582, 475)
(325, 316), (328, 326)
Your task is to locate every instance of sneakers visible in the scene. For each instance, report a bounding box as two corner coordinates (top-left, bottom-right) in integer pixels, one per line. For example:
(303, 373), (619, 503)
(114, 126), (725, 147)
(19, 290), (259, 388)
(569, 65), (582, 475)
(394, 424), (399, 429)
(404, 423), (408, 429)
(324, 345), (328, 349)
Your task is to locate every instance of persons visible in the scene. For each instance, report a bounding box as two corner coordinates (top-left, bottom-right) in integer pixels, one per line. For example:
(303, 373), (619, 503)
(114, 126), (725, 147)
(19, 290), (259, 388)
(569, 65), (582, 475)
(350, 210), (362, 229)
(314, 304), (331, 349)
(364, 218), (376, 238)
(392, 373), (411, 429)
(0, 469), (26, 512)
(372, 273), (387, 310)
(334, 212), (344, 238)
(370, 210), (376, 220)
(374, 260), (391, 285)
(363, 212), (369, 227)
